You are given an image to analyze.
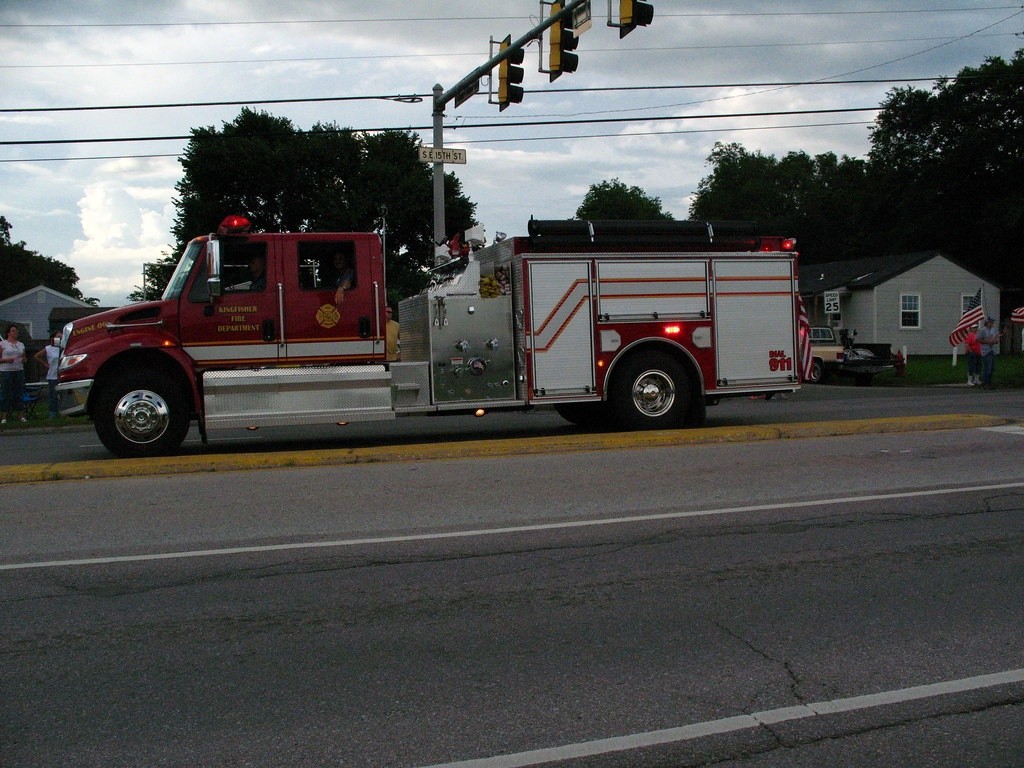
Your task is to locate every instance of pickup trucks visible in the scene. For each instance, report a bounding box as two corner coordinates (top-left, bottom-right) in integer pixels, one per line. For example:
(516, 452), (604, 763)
(804, 325), (846, 385)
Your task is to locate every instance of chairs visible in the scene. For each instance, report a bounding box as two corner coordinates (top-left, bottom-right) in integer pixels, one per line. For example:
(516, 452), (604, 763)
(22, 385), (41, 420)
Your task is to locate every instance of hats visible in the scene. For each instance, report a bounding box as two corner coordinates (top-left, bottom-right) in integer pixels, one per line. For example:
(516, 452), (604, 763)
(971, 323), (978, 328)
(984, 318), (995, 325)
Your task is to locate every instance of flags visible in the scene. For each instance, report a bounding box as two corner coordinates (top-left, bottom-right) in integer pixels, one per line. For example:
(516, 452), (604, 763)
(948, 287), (987, 347)
(1010, 307), (1024, 322)
(798, 295), (816, 380)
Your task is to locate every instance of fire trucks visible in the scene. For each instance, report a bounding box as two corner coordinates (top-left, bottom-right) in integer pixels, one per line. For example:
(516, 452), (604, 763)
(56, 216), (814, 461)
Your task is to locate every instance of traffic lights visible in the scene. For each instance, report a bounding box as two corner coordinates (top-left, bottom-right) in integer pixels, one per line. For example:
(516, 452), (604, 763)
(550, 1), (581, 82)
(499, 34), (526, 109)
(618, 0), (656, 38)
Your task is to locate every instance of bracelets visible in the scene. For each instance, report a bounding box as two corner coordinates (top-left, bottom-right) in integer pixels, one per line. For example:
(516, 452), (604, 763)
(340, 284), (347, 290)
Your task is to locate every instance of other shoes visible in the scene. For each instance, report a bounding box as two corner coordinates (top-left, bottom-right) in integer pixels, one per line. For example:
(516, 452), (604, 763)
(49, 415), (55, 420)
(989, 386), (995, 389)
(20, 418), (26, 422)
(1, 419), (7, 423)
(981, 385), (987, 390)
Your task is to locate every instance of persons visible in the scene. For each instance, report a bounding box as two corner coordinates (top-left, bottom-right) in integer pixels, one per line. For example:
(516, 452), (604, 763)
(0, 324), (27, 424)
(34, 331), (68, 420)
(386, 307), (402, 362)
(974, 316), (1000, 391)
(964, 324), (982, 386)
(244, 257), (266, 292)
(331, 253), (356, 305)
(751, 392), (789, 401)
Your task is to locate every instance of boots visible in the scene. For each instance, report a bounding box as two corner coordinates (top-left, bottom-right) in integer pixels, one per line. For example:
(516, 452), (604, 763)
(968, 374), (982, 386)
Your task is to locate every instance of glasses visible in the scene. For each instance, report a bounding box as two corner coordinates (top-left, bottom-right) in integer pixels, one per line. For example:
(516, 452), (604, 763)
(385, 311), (394, 314)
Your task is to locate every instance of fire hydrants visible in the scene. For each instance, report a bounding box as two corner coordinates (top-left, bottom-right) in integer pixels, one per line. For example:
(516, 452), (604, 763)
(892, 350), (907, 377)
